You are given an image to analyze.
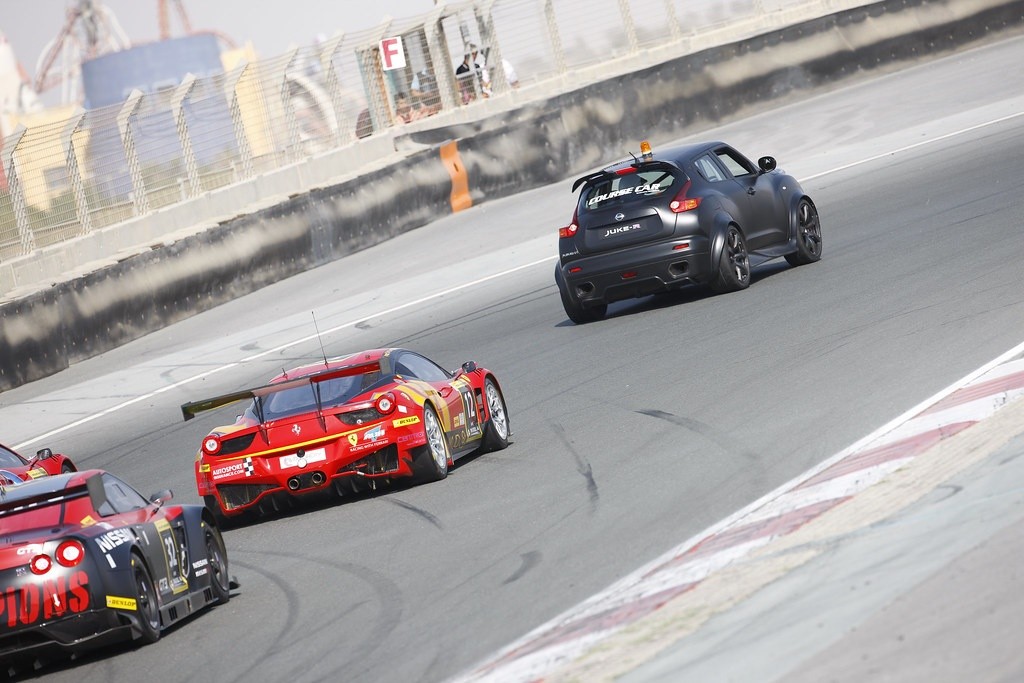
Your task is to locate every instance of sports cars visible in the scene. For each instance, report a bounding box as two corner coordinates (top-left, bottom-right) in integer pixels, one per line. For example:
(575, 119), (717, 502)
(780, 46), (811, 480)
(0, 469), (241, 683)
(0, 442), (79, 490)
(194, 347), (513, 532)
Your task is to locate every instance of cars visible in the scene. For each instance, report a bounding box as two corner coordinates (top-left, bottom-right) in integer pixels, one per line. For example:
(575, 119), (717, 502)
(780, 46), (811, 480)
(554, 141), (822, 324)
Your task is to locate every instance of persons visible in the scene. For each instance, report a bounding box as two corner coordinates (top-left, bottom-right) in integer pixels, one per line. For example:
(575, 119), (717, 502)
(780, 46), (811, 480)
(410, 64), (440, 106)
(455, 43), (520, 104)
(394, 92), (416, 123)
(618, 175), (642, 193)
